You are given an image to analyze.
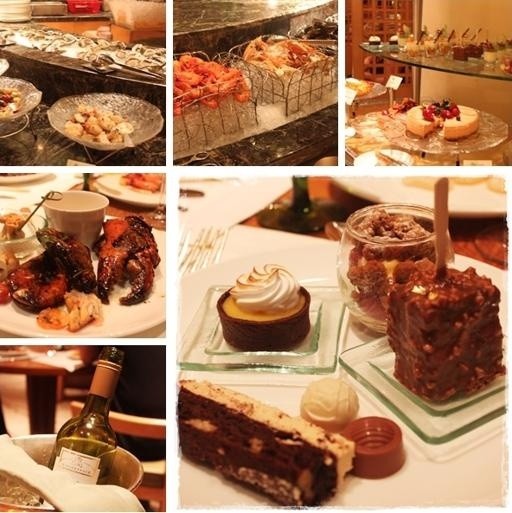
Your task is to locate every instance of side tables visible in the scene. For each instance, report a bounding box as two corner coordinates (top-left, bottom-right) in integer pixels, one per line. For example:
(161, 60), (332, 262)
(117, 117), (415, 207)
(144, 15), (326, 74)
(0, 345), (96, 435)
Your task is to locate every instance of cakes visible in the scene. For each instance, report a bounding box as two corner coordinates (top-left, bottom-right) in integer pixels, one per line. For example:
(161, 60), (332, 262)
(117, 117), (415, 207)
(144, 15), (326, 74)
(404, 96), (481, 141)
(216, 262), (312, 351)
(386, 258), (506, 403)
(179, 378), (356, 509)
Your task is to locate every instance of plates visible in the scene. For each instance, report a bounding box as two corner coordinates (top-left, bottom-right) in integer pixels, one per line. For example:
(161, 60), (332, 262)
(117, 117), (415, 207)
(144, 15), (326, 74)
(336, 334), (506, 445)
(334, 178), (506, 221)
(180, 216), (504, 505)
(1, 75), (44, 124)
(179, 281), (346, 379)
(47, 92), (165, 151)
(0, 228), (168, 337)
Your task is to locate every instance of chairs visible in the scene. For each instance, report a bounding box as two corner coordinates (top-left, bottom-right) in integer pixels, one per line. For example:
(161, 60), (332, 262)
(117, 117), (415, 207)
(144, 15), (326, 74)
(70, 399), (166, 512)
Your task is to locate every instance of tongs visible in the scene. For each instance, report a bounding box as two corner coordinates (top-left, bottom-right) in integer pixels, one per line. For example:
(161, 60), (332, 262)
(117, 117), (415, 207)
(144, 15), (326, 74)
(92, 53), (165, 83)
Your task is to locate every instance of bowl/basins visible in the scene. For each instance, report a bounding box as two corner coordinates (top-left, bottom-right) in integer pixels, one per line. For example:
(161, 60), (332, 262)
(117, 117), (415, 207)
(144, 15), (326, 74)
(1, 208), (37, 247)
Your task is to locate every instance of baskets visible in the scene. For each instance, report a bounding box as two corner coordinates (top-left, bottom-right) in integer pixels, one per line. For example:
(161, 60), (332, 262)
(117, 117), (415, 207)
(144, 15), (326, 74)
(175, 35), (338, 153)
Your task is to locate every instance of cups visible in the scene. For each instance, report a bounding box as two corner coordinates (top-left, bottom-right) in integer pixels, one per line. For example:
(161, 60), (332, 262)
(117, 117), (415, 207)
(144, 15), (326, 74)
(405, 39), (465, 58)
(482, 44), (512, 69)
(335, 202), (454, 338)
(39, 191), (110, 251)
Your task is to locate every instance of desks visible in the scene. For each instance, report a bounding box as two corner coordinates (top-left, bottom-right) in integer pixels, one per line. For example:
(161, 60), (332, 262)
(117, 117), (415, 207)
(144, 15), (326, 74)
(346, 103), (511, 166)
(359, 42), (512, 80)
(346, 81), (386, 118)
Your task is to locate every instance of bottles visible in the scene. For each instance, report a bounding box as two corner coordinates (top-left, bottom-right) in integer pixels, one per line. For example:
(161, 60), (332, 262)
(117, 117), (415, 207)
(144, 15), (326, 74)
(48, 346), (127, 479)
